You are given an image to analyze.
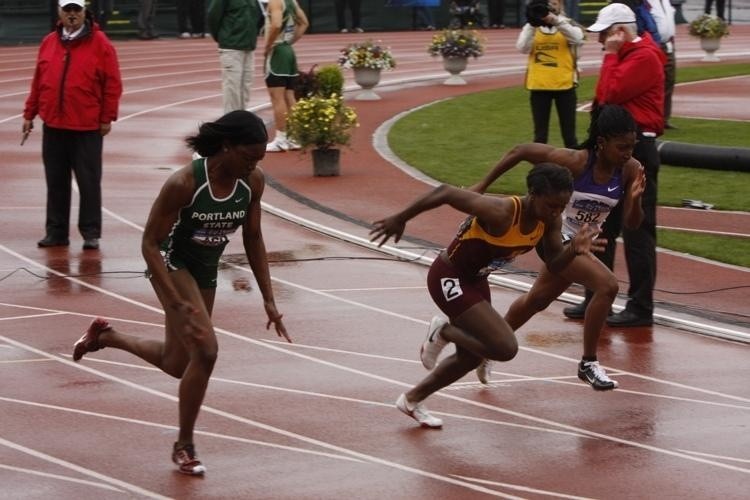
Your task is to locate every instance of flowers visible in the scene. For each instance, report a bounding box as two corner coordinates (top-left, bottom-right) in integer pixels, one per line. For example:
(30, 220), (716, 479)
(425, 28), (486, 60)
(281, 89), (360, 147)
(335, 36), (395, 70)
(685, 13), (729, 38)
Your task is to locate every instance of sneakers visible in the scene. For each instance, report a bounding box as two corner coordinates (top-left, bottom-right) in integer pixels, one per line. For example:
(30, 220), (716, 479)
(172, 441), (206, 475)
(419, 315), (450, 371)
(287, 138), (302, 150)
(562, 300), (612, 319)
(72, 316), (113, 361)
(606, 310), (653, 327)
(476, 357), (493, 385)
(265, 139), (288, 152)
(395, 391), (443, 427)
(577, 359), (620, 391)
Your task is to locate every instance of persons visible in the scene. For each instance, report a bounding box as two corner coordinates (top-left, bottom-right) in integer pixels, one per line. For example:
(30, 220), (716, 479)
(263, 1), (309, 152)
(73, 111), (292, 475)
(519, 2), (724, 31)
(608, 0), (668, 54)
(459, 104), (645, 390)
(206, 0), (265, 117)
(369, 163), (608, 429)
(562, 4), (665, 328)
(401, 1), (507, 31)
(338, 1), (365, 33)
(26, 0), (122, 250)
(90, 0), (212, 41)
(644, 0), (677, 129)
(516, 0), (584, 150)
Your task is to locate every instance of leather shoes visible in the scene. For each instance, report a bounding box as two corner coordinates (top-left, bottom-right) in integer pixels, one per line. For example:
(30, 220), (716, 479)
(82, 239), (100, 250)
(37, 236), (70, 247)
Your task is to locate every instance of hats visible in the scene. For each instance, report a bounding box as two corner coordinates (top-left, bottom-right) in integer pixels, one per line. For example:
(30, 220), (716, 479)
(583, 3), (637, 33)
(58, 0), (86, 8)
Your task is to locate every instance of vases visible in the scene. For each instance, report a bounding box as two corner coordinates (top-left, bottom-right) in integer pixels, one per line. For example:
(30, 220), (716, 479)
(700, 35), (718, 62)
(353, 67), (381, 101)
(443, 56), (466, 85)
(311, 148), (339, 177)
